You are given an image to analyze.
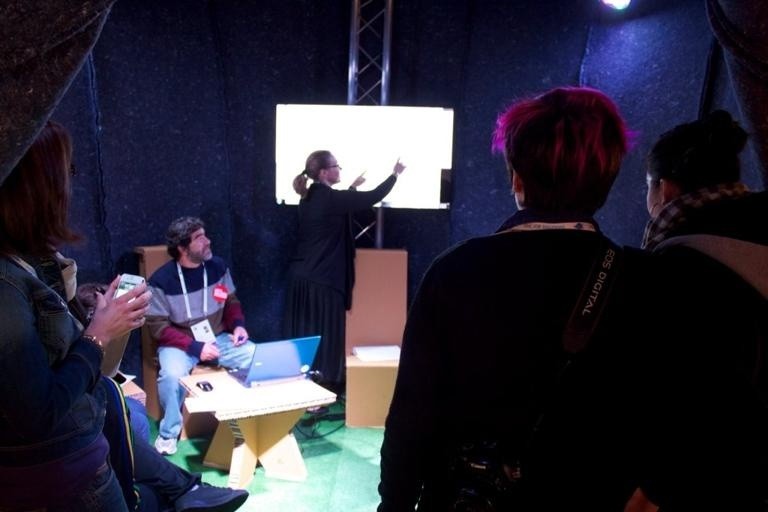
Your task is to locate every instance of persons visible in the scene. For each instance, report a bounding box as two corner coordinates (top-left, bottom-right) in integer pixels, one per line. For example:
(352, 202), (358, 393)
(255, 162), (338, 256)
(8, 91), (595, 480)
(278, 150), (407, 397)
(375, 83), (768, 511)
(1, 117), (249, 512)
(143, 214), (256, 456)
(640, 102), (768, 299)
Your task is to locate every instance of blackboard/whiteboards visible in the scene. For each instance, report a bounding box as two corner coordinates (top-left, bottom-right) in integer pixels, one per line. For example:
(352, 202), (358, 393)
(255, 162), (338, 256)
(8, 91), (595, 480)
(274, 103), (454, 210)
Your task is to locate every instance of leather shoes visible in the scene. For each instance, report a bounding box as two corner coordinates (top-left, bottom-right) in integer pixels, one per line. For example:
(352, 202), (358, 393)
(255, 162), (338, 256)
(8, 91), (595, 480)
(306, 406), (328, 413)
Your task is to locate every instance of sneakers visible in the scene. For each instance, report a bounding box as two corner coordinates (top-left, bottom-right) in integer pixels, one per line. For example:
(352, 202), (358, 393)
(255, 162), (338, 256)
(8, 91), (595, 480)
(174, 480), (249, 511)
(154, 434), (177, 455)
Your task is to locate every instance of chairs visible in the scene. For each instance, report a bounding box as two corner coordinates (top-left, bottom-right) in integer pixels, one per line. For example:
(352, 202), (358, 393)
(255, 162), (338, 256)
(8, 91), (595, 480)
(343, 249), (410, 429)
(135, 244), (220, 441)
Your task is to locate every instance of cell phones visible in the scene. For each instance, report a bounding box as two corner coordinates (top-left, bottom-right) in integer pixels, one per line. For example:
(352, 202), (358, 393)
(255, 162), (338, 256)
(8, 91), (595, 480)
(112, 272), (145, 302)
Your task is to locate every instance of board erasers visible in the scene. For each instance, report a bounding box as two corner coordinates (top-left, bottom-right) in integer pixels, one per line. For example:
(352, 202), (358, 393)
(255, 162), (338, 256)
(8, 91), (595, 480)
(440, 169), (452, 203)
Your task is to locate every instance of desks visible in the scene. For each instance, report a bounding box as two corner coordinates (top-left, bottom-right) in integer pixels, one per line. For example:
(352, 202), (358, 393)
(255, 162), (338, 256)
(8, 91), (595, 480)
(177, 368), (338, 490)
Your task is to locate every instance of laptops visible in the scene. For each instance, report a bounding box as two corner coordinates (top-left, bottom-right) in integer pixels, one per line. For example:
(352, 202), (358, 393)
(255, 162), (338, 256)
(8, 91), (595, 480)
(227, 334), (322, 387)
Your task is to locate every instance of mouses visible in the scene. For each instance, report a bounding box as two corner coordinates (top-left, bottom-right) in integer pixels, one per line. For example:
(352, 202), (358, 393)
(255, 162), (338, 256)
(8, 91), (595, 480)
(196, 379), (214, 393)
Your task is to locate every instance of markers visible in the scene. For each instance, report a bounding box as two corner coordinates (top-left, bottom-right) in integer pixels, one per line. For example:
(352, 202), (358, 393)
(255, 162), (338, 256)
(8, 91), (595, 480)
(228, 333), (243, 341)
